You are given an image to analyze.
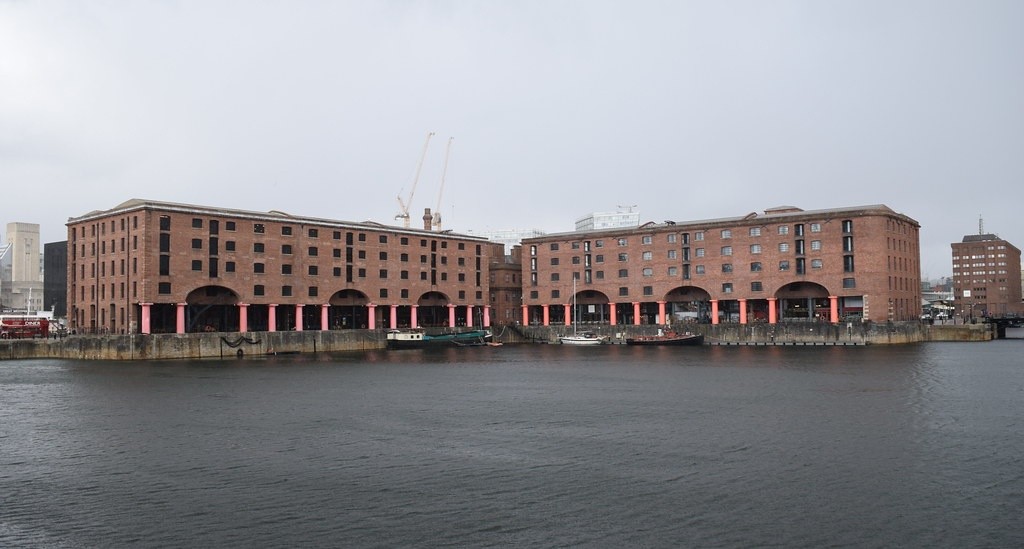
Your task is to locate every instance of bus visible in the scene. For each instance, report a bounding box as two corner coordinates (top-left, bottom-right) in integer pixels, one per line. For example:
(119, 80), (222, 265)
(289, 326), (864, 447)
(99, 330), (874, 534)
(1, 315), (49, 338)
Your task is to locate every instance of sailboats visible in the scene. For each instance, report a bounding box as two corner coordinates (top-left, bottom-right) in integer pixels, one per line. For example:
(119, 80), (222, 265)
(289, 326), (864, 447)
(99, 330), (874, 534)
(560, 274), (607, 346)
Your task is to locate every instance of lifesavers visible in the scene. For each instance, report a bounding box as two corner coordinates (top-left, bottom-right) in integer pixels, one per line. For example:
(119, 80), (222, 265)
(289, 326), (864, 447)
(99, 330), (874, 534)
(237, 349), (243, 357)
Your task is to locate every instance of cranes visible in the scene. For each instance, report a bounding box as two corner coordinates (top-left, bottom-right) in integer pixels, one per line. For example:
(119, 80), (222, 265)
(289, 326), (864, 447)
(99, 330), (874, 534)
(397, 131), (435, 229)
(432, 137), (454, 231)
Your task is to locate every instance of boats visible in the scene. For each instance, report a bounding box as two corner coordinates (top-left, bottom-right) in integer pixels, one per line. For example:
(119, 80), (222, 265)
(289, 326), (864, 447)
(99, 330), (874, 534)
(425, 328), (492, 346)
(387, 329), (423, 347)
(625, 328), (704, 346)
